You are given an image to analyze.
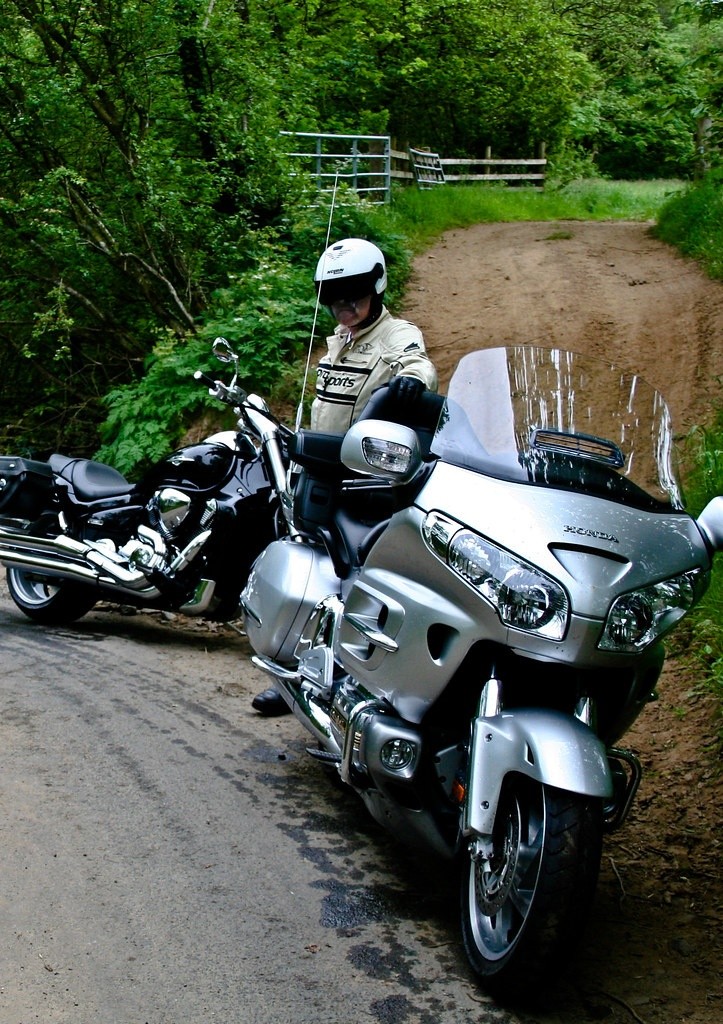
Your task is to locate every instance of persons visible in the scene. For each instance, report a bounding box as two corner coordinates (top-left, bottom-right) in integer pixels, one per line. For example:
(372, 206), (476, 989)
(252, 239), (439, 717)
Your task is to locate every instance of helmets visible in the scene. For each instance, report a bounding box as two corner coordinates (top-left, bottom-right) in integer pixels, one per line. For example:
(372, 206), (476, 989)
(313, 238), (388, 306)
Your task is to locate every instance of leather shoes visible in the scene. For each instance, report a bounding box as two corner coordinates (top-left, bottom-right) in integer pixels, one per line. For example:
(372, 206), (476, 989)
(252, 685), (289, 715)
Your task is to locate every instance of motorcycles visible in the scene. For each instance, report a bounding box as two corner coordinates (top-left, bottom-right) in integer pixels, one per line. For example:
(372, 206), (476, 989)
(240, 341), (723, 1006)
(1, 334), (301, 627)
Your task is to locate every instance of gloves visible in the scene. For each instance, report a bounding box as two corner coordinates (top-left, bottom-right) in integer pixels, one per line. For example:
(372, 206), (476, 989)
(371, 374), (425, 407)
